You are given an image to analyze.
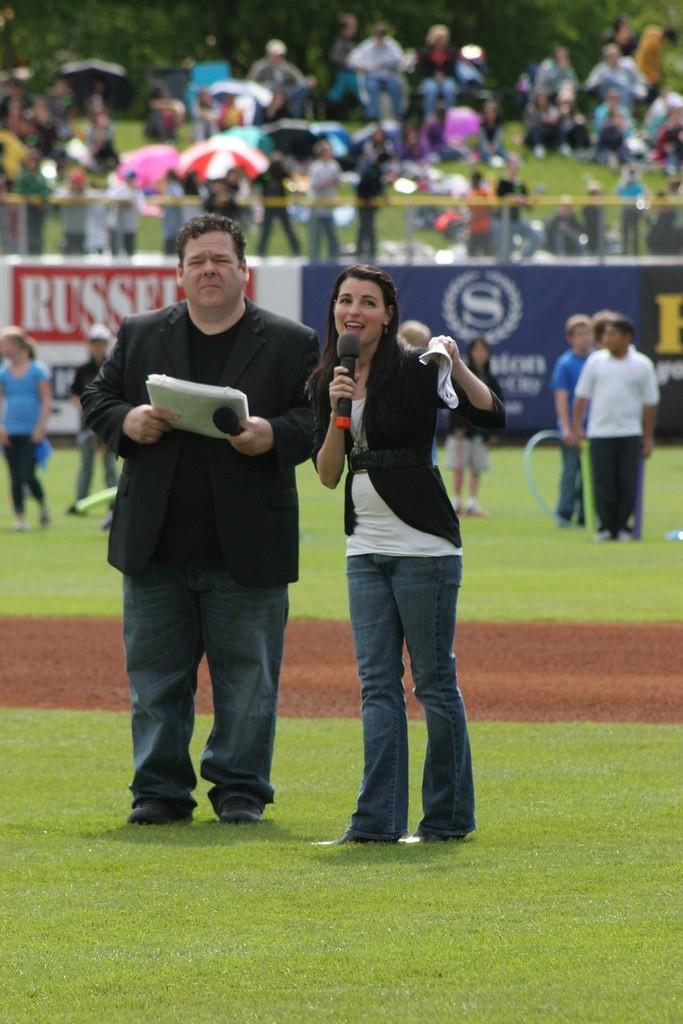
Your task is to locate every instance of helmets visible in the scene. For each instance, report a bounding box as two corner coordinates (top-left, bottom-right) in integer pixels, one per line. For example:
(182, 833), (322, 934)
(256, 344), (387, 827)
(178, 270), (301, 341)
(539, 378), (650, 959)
(87, 323), (111, 341)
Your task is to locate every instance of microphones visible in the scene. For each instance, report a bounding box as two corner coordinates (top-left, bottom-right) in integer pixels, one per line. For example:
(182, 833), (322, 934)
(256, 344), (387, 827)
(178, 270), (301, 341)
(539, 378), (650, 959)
(213, 405), (243, 436)
(334, 334), (361, 430)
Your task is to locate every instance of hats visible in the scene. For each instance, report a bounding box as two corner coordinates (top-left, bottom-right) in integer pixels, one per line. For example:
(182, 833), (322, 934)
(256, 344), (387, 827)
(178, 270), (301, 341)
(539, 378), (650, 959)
(124, 168), (136, 178)
(665, 91), (683, 106)
(71, 172), (85, 182)
(266, 39), (287, 54)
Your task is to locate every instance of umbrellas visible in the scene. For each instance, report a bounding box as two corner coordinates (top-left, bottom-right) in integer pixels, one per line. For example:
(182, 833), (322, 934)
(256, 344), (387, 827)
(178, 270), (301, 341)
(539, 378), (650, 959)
(208, 80), (272, 109)
(444, 105), (483, 138)
(119, 142), (181, 189)
(60, 58), (135, 109)
(175, 134), (271, 182)
(263, 118), (319, 162)
(220, 125), (273, 154)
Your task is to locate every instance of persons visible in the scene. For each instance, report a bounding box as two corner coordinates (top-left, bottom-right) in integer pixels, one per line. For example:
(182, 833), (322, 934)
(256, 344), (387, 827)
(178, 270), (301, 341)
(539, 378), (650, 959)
(0, 14), (682, 261)
(66, 323), (120, 515)
(0, 328), (53, 531)
(81, 214), (320, 822)
(554, 309), (659, 542)
(305, 265), (503, 845)
(447, 340), (503, 518)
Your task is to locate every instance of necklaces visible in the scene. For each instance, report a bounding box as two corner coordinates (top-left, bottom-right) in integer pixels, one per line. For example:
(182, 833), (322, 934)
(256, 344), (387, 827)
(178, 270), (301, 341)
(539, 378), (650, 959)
(349, 411), (363, 457)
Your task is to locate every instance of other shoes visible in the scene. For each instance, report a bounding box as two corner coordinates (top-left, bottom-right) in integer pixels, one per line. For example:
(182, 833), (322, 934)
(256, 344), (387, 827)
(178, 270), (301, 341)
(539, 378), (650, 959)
(452, 495), (460, 512)
(13, 520), (26, 531)
(69, 505), (83, 515)
(465, 499), (490, 517)
(593, 526), (614, 541)
(128, 799), (194, 825)
(617, 525), (635, 540)
(40, 515), (48, 529)
(311, 834), (399, 846)
(396, 829), (466, 843)
(222, 799), (261, 823)
